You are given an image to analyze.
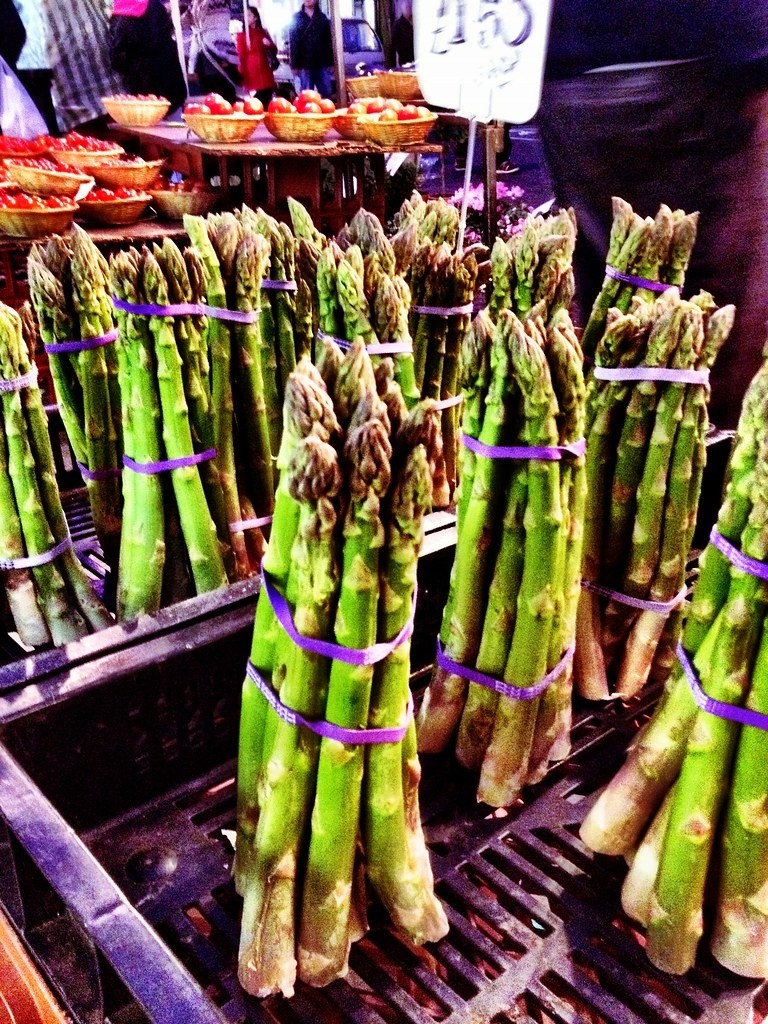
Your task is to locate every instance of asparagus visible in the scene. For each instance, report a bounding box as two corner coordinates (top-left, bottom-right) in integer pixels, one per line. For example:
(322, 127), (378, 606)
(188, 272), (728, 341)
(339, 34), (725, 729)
(0, 189), (768, 1001)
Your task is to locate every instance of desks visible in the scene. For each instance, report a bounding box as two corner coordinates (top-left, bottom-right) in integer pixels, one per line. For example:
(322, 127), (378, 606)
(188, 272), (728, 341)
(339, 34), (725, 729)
(0, 207), (188, 405)
(109, 122), (443, 237)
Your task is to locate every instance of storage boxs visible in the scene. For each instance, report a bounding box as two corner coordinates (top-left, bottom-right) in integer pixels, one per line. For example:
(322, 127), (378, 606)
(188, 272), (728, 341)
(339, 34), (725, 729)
(0, 426), (768, 1023)
(0, 404), (712, 712)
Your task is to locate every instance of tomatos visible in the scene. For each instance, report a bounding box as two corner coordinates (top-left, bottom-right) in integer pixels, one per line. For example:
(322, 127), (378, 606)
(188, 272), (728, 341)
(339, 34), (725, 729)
(0, 89), (433, 210)
